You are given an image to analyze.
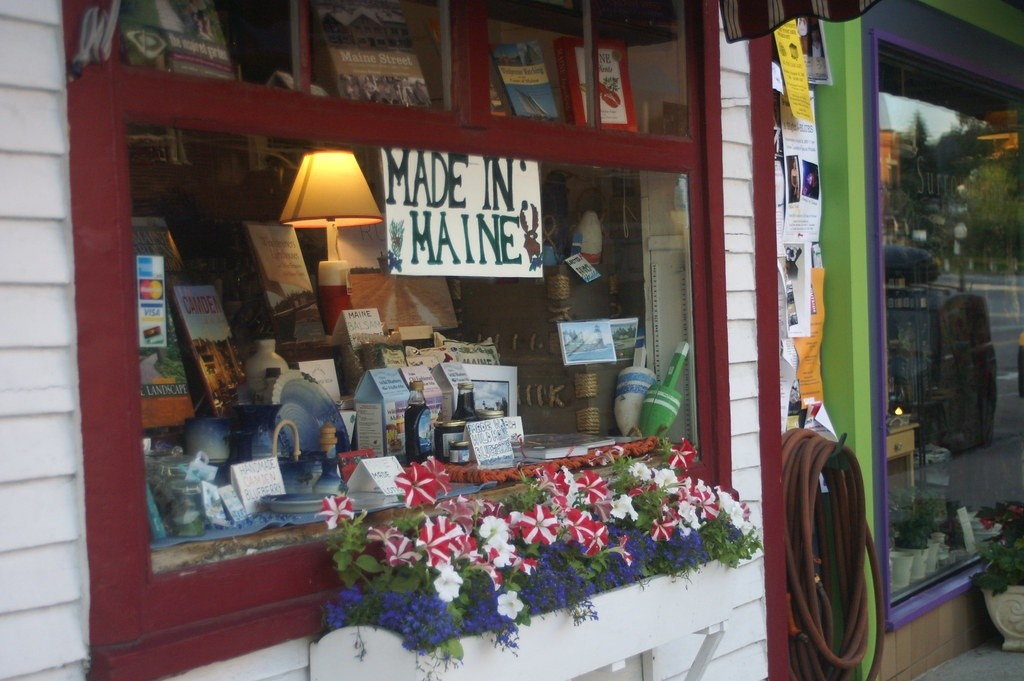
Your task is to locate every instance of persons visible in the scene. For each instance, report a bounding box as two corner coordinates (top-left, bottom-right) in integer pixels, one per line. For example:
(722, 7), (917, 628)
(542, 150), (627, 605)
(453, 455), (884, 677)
(889, 349), (911, 400)
(785, 248), (798, 280)
(345, 74), (415, 106)
(790, 161), (798, 203)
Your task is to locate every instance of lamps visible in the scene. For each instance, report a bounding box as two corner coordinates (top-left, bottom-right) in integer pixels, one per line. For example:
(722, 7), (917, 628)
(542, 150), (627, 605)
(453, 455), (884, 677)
(278, 151), (384, 334)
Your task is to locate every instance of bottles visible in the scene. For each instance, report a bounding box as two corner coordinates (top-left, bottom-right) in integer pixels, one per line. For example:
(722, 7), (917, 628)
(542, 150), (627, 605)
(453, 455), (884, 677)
(262, 367), (281, 406)
(452, 382), (479, 423)
(404, 381), (431, 464)
(313, 421), (349, 500)
(243, 338), (289, 402)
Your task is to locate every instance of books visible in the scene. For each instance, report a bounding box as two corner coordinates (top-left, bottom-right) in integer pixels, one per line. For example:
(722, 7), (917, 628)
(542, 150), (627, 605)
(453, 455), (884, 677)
(327, 211), (459, 333)
(171, 286), (250, 417)
(312, 0), (431, 106)
(488, 39), (558, 118)
(242, 222), (326, 346)
(116, 0), (234, 81)
(555, 36), (637, 132)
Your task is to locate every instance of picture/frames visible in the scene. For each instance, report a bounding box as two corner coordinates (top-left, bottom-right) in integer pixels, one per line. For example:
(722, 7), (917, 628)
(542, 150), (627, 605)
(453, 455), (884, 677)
(463, 364), (518, 417)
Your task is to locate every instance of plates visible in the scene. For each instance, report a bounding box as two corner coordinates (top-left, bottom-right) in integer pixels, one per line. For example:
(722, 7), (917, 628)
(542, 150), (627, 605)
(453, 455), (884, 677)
(272, 370), (350, 459)
(260, 493), (337, 513)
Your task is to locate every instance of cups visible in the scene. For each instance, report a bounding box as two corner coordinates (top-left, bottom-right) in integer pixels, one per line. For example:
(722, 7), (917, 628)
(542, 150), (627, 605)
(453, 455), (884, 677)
(184, 417), (231, 463)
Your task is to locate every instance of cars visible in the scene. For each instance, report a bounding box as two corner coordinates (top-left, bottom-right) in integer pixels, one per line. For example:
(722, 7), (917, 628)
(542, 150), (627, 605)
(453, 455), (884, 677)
(883, 245), (998, 451)
(1017, 333), (1024, 400)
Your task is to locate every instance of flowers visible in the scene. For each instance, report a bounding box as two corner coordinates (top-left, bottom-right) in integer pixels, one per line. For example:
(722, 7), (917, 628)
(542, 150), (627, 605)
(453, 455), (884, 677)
(314, 437), (762, 681)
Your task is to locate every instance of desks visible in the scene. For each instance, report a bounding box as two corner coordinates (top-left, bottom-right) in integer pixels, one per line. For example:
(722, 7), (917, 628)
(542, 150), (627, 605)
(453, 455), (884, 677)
(886, 423), (920, 506)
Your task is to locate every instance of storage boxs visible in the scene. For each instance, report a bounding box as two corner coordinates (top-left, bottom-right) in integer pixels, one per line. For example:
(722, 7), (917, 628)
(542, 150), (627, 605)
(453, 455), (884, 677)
(397, 365), (442, 455)
(430, 362), (471, 423)
(354, 367), (410, 464)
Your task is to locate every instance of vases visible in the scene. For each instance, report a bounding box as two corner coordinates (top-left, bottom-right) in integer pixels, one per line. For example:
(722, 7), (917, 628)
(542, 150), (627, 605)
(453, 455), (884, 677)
(309, 560), (732, 681)
